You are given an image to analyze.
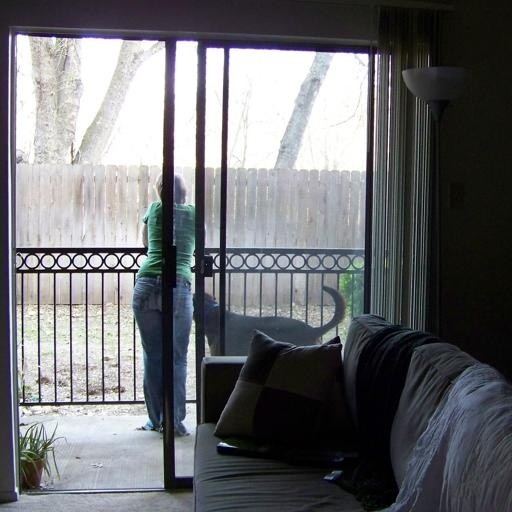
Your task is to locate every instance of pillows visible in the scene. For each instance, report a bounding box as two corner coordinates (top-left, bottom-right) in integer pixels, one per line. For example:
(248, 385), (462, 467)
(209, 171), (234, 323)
(213, 327), (343, 469)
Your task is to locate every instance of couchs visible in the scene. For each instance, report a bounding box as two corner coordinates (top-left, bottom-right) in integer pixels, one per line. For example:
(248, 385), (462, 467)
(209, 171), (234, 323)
(195, 316), (512, 511)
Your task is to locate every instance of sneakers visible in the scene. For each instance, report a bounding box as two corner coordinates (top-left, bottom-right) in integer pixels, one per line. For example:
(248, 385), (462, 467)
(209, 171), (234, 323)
(147, 420), (189, 437)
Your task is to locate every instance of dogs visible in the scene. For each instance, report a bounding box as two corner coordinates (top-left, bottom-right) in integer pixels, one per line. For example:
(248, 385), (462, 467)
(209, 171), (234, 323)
(193, 284), (345, 356)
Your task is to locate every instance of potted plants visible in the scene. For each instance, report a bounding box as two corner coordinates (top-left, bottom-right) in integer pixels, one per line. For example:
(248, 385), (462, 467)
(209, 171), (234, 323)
(19, 421), (66, 494)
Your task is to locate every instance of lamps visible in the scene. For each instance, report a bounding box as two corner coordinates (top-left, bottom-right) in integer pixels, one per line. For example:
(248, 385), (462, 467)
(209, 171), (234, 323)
(399, 66), (475, 337)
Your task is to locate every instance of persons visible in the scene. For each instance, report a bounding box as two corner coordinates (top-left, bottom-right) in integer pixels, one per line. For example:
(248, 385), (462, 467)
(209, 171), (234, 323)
(129, 171), (206, 437)
